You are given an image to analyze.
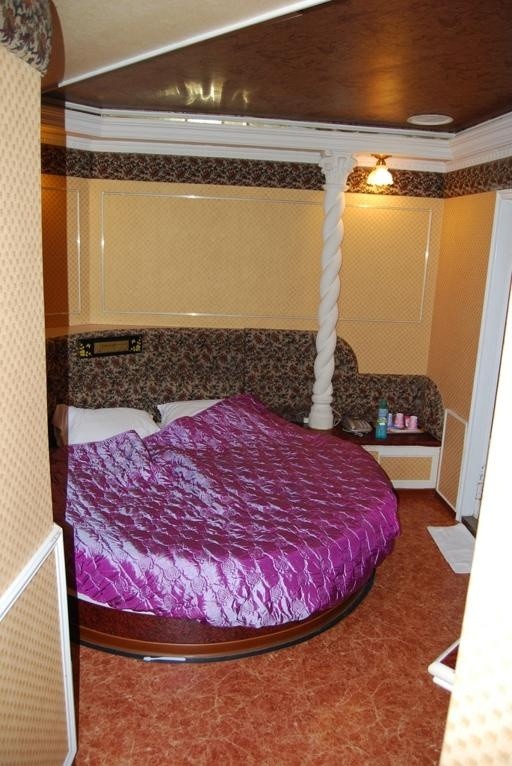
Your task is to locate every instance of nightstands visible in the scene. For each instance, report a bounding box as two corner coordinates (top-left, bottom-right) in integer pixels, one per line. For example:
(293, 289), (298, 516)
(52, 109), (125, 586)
(293, 421), (440, 490)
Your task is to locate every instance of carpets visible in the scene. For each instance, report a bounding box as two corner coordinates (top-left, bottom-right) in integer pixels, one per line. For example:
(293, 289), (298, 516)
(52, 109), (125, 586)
(426, 522), (477, 574)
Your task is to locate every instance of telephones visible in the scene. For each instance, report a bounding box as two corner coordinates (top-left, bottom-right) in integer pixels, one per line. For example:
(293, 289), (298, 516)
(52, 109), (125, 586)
(342, 417), (373, 437)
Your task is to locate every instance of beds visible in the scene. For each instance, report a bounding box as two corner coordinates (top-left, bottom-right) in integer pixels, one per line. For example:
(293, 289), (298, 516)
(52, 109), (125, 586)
(45, 324), (401, 666)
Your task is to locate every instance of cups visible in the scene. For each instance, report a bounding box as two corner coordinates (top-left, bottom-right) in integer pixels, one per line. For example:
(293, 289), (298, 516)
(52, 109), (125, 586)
(394, 413), (404, 429)
(405, 416), (417, 430)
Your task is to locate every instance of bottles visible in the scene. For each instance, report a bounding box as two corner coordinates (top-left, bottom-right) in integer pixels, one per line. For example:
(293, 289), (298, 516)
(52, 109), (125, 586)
(377, 400), (388, 418)
(375, 418), (387, 440)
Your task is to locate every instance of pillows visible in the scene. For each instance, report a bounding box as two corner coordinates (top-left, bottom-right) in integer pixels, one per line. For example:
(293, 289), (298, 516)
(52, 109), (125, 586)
(155, 399), (229, 429)
(52, 402), (163, 447)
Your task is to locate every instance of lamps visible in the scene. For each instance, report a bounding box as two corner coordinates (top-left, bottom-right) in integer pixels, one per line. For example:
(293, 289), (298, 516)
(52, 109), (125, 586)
(364, 151), (398, 186)
